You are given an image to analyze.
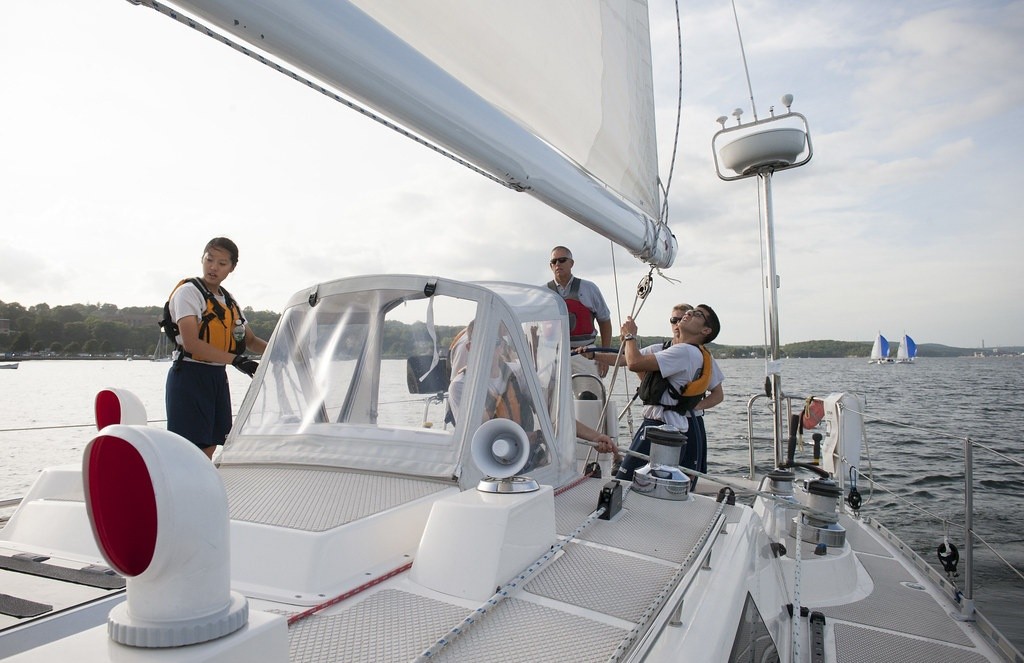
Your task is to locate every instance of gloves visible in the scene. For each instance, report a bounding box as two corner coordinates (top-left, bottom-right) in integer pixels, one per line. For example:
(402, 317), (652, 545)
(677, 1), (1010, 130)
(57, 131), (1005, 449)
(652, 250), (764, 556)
(232, 355), (260, 378)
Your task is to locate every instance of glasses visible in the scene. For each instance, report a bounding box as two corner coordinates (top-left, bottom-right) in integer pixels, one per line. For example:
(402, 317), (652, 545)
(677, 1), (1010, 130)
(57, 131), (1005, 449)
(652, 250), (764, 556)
(685, 310), (708, 322)
(550, 257), (569, 264)
(670, 317), (682, 325)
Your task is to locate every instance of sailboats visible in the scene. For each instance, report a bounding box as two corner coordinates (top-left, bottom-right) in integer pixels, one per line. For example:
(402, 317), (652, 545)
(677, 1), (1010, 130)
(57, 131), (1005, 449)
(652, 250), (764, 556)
(869, 329), (896, 364)
(0, 2), (1024, 663)
(895, 330), (918, 364)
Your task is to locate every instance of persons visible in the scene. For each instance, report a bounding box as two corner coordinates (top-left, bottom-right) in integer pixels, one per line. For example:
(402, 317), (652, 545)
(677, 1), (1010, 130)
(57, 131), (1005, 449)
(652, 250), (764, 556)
(444, 317), (619, 476)
(615, 303), (725, 492)
(543, 246), (612, 400)
(158, 238), (268, 460)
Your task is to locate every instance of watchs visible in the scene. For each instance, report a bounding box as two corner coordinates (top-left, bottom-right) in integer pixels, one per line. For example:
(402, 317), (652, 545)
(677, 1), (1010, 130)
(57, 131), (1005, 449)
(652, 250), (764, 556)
(625, 333), (636, 340)
